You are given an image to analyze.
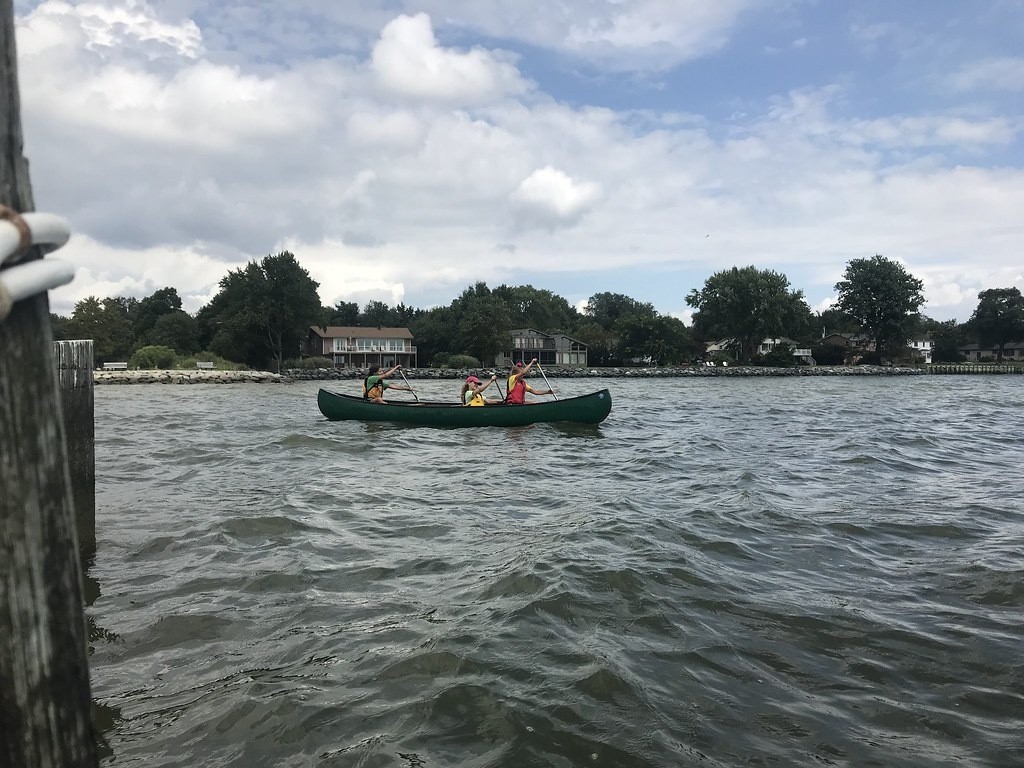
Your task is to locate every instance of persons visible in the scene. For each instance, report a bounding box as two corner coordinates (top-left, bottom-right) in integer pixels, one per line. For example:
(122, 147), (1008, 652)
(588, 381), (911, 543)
(363, 363), (411, 404)
(461, 376), (504, 406)
(505, 358), (553, 405)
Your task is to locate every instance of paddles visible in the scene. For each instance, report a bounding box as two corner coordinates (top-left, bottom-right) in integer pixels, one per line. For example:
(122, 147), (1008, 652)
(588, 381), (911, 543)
(494, 379), (505, 401)
(535, 360), (560, 401)
(398, 368), (419, 402)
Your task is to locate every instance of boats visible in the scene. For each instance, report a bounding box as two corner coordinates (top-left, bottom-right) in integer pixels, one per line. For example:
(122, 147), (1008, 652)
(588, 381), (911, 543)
(318, 388), (613, 423)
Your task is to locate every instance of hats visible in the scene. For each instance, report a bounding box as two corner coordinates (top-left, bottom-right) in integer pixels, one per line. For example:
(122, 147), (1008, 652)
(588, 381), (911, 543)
(465, 376), (482, 386)
